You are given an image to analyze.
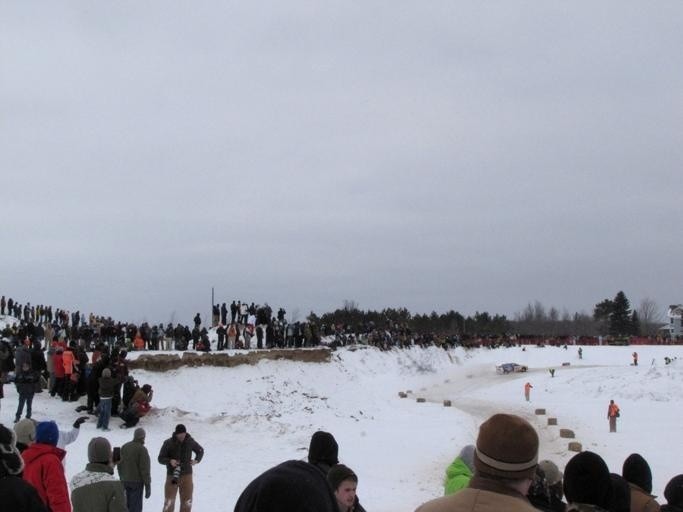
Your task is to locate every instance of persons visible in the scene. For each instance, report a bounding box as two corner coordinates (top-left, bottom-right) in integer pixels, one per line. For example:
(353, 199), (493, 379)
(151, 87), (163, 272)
(578, 347), (582, 359)
(1, 416), (204, 512)
(607, 400), (619, 433)
(524, 382), (533, 401)
(234, 430), (367, 512)
(153, 321), (682, 353)
(632, 352), (638, 365)
(193, 298), (287, 321)
(1, 295), (152, 432)
(415, 413), (683, 512)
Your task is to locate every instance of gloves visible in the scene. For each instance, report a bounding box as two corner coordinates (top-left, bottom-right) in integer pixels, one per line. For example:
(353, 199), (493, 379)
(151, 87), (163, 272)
(73, 416), (89, 428)
(145, 484), (151, 498)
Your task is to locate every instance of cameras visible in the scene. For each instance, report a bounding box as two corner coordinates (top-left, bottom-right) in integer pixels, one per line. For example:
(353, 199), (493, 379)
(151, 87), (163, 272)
(172, 461), (183, 485)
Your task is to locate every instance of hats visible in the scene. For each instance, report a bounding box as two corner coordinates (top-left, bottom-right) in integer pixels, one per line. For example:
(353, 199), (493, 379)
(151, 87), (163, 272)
(102, 368), (111, 378)
(664, 474), (682, 499)
(307, 431), (339, 464)
(327, 464), (355, 494)
(623, 453), (652, 495)
(538, 459), (563, 486)
(13, 418), (59, 447)
(473, 414), (539, 479)
(0, 424), (25, 475)
(88, 437), (111, 463)
(134, 428), (145, 440)
(564, 450), (630, 512)
(459, 445), (476, 473)
(175, 424), (186, 434)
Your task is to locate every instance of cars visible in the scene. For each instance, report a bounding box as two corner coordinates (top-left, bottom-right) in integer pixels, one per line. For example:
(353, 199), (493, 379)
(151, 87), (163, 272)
(494, 363), (528, 375)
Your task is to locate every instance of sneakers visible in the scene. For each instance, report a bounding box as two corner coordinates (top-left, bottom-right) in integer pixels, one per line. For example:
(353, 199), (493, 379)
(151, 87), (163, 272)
(97, 422), (126, 432)
